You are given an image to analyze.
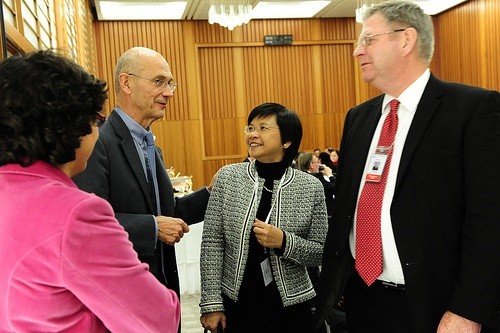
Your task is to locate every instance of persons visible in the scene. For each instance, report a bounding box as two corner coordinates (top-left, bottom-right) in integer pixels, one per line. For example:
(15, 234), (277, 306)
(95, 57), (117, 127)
(243, 146), (339, 209)
(71, 46), (217, 333)
(322, 0), (500, 333)
(199, 103), (328, 333)
(0, 48), (181, 333)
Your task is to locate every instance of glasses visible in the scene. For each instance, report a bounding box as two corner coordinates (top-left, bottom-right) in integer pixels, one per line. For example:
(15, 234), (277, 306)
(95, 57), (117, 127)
(243, 125), (280, 136)
(312, 161), (321, 163)
(128, 73), (177, 93)
(94, 111), (106, 128)
(354, 28), (406, 47)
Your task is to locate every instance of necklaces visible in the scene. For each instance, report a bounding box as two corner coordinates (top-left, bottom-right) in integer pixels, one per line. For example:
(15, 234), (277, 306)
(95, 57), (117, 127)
(263, 185), (273, 192)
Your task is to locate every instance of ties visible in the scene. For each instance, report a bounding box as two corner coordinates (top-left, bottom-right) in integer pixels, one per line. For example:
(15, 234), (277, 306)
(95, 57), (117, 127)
(353, 99), (399, 287)
(145, 133), (162, 218)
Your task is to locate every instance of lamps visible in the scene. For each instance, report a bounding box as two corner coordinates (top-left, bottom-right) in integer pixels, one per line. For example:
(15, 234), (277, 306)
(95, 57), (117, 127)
(356, 0), (376, 23)
(208, 0), (253, 30)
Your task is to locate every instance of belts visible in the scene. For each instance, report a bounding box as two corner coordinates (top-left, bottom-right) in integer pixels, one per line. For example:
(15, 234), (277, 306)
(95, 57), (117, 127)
(374, 280), (406, 292)
